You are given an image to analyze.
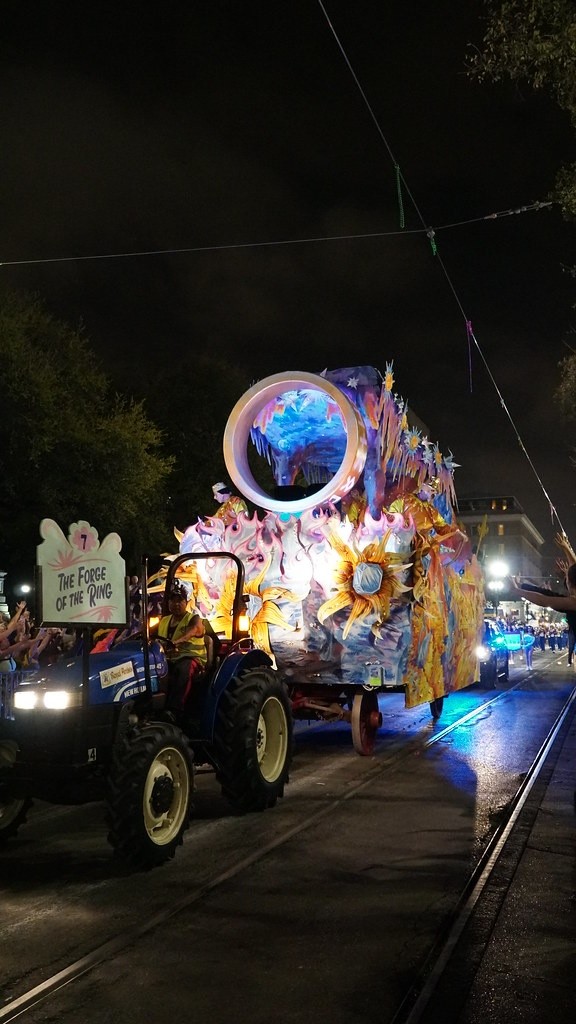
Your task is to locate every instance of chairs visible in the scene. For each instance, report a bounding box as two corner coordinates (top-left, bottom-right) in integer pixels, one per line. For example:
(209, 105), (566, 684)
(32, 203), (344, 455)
(192, 635), (213, 689)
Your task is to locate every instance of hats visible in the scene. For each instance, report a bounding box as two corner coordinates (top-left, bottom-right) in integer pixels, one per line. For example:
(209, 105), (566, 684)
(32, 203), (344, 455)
(168, 589), (187, 600)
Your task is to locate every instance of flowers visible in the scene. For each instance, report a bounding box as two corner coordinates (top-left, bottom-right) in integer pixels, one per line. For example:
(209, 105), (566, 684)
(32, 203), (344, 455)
(139, 360), (461, 670)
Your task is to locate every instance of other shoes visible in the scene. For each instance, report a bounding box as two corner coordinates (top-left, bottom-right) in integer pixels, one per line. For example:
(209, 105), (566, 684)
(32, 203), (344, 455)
(166, 710), (179, 725)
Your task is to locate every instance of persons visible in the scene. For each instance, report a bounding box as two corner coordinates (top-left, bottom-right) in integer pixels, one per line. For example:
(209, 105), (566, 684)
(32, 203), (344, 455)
(130, 576), (138, 590)
(503, 532), (576, 670)
(0, 600), (162, 720)
(158, 587), (208, 719)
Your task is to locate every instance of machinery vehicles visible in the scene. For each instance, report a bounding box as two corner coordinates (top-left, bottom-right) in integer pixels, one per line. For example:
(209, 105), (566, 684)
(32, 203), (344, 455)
(0, 534), (451, 864)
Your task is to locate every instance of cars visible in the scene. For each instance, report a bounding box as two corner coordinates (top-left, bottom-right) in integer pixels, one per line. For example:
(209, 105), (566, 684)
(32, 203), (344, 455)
(475, 616), (510, 690)
